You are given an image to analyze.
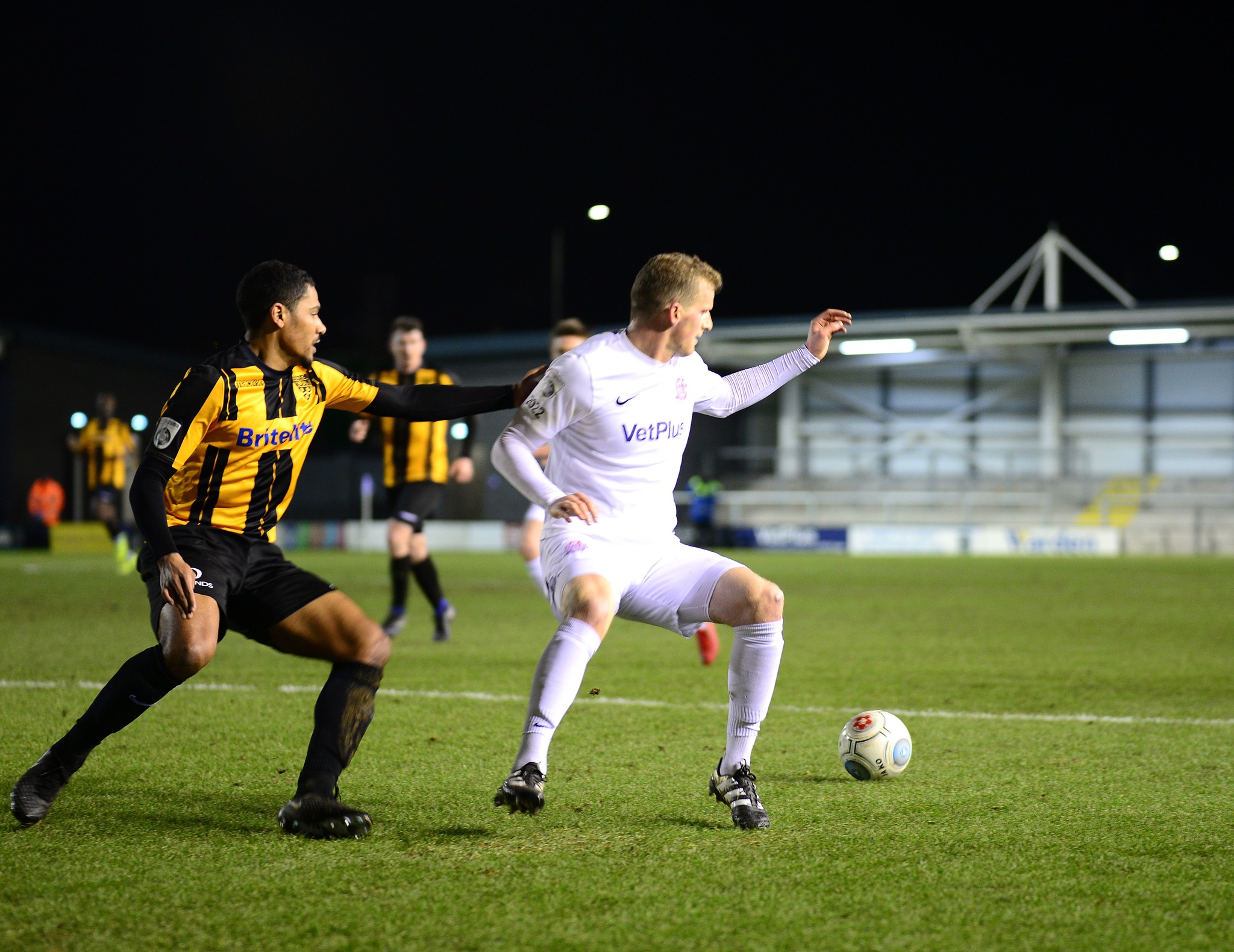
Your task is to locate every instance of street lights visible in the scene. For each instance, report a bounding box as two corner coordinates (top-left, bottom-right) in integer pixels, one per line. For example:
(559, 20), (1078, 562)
(546, 203), (610, 320)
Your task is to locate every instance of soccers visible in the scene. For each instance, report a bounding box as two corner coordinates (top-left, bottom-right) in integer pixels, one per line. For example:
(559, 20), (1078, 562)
(837, 709), (913, 782)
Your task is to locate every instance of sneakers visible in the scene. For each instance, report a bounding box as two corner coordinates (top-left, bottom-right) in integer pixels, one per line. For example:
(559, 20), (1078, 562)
(382, 610), (408, 637)
(276, 792), (372, 839)
(116, 551), (138, 577)
(12, 743), (68, 824)
(497, 762), (547, 814)
(705, 758), (770, 831)
(435, 603), (454, 639)
(114, 534), (130, 564)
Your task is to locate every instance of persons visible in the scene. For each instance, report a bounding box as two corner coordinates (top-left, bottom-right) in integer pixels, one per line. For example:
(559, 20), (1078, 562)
(491, 251), (852, 828)
(27, 476), (66, 526)
(10, 259), (549, 841)
(82, 391), (140, 577)
(347, 316), (479, 643)
(685, 467), (722, 546)
(519, 314), (586, 603)
